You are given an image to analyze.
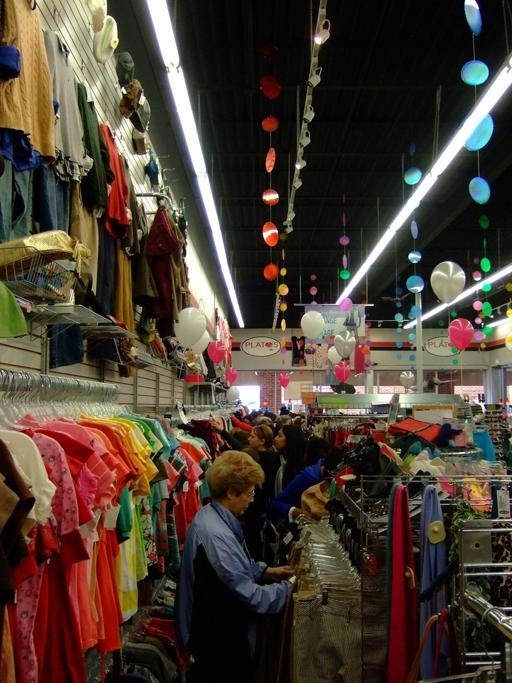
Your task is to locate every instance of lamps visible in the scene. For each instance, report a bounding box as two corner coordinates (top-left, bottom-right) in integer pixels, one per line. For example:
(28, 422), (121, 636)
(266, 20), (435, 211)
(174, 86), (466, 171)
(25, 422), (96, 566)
(335, 0), (512, 329)
(145, 0), (247, 328)
(281, 8), (332, 236)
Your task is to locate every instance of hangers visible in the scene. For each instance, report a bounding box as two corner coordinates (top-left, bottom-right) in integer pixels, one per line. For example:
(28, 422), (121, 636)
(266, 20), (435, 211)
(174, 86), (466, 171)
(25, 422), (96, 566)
(419, 607), (508, 683)
(0, 368), (126, 434)
(446, 449), (479, 464)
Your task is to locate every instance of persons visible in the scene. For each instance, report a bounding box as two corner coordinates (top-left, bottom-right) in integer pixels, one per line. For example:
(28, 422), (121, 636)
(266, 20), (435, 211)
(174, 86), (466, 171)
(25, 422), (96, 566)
(209, 407), (350, 563)
(174, 449), (299, 682)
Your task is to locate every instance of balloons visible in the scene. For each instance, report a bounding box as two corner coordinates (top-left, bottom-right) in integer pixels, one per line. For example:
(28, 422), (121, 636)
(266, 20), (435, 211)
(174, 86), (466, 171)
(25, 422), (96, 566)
(301, 311), (324, 341)
(225, 368), (238, 386)
(327, 330), (356, 384)
(448, 318), (475, 352)
(400, 371), (415, 390)
(430, 260), (466, 305)
(173, 307), (207, 350)
(189, 329), (210, 355)
(207, 341), (225, 366)
(279, 372), (290, 388)
